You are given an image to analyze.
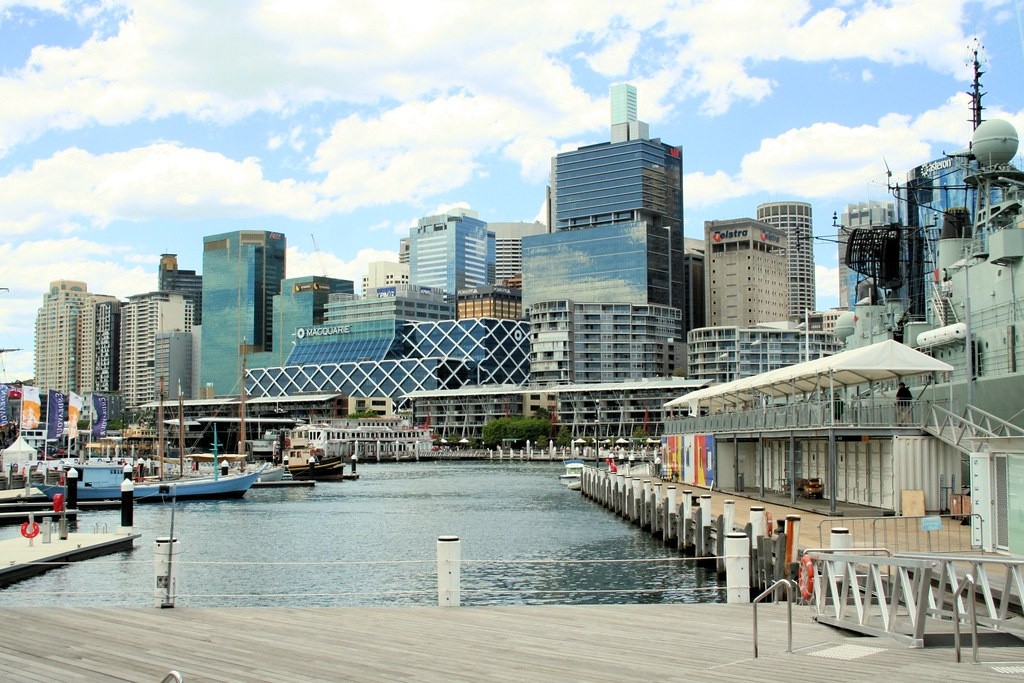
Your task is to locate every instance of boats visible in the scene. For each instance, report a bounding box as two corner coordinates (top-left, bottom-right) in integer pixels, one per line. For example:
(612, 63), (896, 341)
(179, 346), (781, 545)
(33, 461), (287, 504)
(559, 458), (584, 490)
(287, 453), (344, 482)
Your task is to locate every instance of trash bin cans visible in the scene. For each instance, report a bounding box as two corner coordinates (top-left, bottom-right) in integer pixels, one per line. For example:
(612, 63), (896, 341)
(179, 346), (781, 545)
(950, 494), (970, 520)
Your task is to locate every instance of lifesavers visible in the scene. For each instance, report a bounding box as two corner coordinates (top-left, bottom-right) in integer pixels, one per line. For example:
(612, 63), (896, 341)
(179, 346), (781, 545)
(21, 521), (39, 538)
(799, 555), (814, 599)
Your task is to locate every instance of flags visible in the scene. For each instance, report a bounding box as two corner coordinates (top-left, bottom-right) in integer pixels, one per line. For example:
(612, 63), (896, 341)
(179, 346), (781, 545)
(0, 384), (106, 439)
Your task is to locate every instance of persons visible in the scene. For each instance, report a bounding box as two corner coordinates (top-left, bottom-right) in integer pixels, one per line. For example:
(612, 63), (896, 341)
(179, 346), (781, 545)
(834, 393), (843, 424)
(896, 382), (913, 428)
(654, 455), (662, 476)
(944, 277), (953, 297)
